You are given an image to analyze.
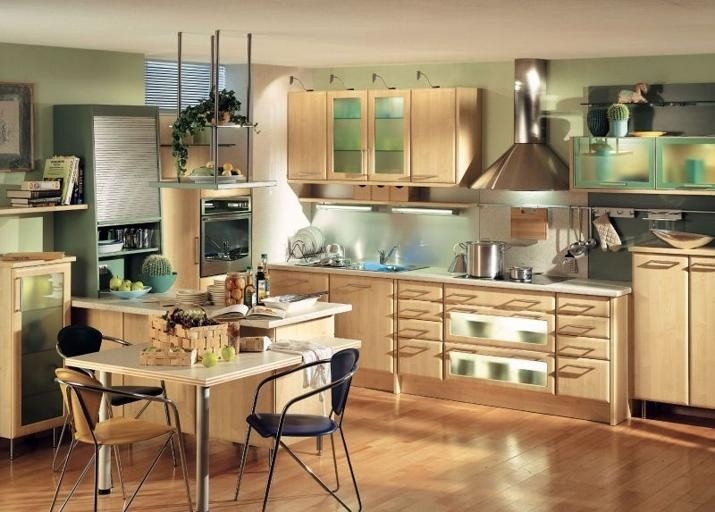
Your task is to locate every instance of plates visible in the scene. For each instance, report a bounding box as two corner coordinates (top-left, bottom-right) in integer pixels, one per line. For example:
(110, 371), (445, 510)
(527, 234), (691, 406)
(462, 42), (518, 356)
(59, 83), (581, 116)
(651, 229), (715, 249)
(175, 274), (227, 306)
(289, 224), (323, 258)
(628, 131), (667, 137)
(110, 286), (153, 299)
(189, 176), (244, 184)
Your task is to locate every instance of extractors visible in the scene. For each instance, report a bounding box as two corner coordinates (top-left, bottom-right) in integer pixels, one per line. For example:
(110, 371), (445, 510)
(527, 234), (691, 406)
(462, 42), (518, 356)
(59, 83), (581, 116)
(467, 58), (569, 190)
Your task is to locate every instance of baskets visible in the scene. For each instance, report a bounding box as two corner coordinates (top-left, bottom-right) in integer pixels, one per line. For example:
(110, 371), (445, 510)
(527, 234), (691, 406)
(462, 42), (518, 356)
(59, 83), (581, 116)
(140, 345), (197, 367)
(151, 315), (240, 362)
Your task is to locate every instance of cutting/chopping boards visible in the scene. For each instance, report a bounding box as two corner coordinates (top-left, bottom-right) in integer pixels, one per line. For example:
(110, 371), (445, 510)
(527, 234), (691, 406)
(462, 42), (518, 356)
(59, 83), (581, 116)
(510, 206), (548, 240)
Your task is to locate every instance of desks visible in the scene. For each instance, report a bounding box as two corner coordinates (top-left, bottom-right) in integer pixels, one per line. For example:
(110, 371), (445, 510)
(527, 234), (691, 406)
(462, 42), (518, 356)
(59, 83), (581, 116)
(63, 336), (362, 510)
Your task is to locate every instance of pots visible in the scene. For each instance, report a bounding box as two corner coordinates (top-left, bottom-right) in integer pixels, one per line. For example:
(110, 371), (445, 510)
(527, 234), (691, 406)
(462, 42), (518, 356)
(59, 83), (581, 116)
(508, 267), (533, 280)
(460, 238), (512, 279)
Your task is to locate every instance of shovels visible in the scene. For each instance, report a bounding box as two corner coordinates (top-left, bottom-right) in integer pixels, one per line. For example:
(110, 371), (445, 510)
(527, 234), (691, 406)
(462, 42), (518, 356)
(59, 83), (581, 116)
(561, 205), (579, 273)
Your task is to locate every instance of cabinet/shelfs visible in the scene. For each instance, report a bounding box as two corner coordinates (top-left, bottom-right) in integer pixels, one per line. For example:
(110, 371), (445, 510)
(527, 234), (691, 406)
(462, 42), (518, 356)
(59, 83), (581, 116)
(51, 104), (165, 299)
(71, 291), (352, 483)
(0, 255), (78, 462)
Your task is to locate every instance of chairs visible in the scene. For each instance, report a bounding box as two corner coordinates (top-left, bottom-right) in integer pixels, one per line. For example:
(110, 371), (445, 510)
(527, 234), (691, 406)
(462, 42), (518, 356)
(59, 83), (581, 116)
(51, 324), (179, 501)
(49, 366), (194, 511)
(231, 348), (363, 512)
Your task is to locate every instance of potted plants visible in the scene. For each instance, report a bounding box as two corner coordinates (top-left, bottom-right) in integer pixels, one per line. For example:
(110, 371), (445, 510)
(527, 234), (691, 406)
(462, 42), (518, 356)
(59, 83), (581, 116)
(171, 87), (260, 183)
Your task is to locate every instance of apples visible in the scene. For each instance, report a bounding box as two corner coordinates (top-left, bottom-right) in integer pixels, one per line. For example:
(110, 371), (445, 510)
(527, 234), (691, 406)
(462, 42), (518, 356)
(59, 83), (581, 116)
(222, 345), (236, 362)
(202, 352), (217, 368)
(131, 281), (144, 291)
(123, 280), (132, 286)
(110, 276), (122, 289)
(119, 283), (131, 291)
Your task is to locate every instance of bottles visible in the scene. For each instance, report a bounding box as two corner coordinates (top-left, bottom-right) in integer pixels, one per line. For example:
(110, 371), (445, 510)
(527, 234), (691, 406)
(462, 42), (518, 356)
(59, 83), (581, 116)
(260, 254), (271, 298)
(225, 271), (247, 306)
(244, 266), (257, 307)
(257, 266), (268, 306)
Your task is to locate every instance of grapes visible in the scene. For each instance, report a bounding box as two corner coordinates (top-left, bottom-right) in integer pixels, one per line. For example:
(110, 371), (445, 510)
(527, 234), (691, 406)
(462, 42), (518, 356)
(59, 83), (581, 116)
(162, 304), (215, 329)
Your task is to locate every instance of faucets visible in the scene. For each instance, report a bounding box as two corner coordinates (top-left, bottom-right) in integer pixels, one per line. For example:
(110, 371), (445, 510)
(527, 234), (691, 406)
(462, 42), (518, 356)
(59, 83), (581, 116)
(377, 243), (398, 264)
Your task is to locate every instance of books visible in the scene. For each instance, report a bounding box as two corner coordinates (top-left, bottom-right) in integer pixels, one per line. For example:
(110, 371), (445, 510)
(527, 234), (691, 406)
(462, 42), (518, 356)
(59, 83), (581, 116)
(208, 304), (287, 324)
(5, 154), (83, 208)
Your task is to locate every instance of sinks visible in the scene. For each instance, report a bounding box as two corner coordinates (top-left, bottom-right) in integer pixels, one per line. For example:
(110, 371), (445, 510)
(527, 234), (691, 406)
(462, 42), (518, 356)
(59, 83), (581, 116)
(342, 260), (431, 273)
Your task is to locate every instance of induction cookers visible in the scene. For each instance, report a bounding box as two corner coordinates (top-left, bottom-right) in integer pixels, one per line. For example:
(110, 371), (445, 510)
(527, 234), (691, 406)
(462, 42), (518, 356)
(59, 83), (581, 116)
(452, 272), (576, 286)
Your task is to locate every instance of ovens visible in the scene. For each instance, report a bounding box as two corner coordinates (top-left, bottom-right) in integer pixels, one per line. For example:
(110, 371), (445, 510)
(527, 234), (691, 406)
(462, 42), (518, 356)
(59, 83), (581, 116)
(200, 212), (252, 277)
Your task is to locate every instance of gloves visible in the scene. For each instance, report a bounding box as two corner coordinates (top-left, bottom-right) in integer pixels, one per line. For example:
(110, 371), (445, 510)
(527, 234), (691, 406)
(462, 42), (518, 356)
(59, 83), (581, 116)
(593, 214), (622, 253)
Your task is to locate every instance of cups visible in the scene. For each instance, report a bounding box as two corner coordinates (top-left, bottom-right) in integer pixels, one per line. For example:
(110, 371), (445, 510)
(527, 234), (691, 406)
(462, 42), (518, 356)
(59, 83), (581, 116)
(684, 159), (703, 185)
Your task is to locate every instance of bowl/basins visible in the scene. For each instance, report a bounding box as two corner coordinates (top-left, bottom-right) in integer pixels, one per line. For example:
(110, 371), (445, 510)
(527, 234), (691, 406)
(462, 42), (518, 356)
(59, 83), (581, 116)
(518, 330), (547, 344)
(518, 368), (539, 383)
(260, 294), (322, 315)
(98, 239), (125, 254)
(466, 321), (493, 339)
(488, 362), (509, 380)
(457, 359), (474, 376)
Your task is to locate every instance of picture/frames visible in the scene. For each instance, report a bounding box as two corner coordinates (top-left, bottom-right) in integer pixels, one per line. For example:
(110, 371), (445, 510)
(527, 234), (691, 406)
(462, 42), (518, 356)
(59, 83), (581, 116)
(1, 81), (36, 173)
